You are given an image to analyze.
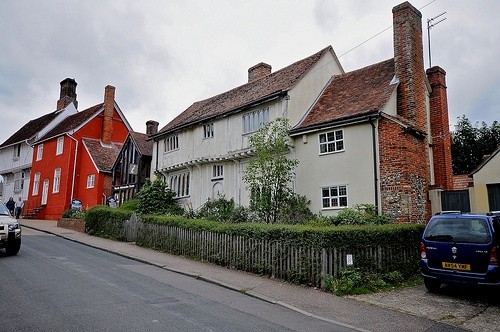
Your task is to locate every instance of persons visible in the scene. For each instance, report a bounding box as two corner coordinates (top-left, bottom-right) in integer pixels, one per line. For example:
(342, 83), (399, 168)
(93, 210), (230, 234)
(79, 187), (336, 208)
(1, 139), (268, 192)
(6, 197), (15, 217)
(15, 196), (24, 219)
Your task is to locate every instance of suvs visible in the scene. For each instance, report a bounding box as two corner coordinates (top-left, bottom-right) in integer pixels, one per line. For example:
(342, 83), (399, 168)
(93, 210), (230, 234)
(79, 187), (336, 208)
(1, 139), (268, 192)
(0, 199), (23, 256)
(420, 211), (499, 296)
